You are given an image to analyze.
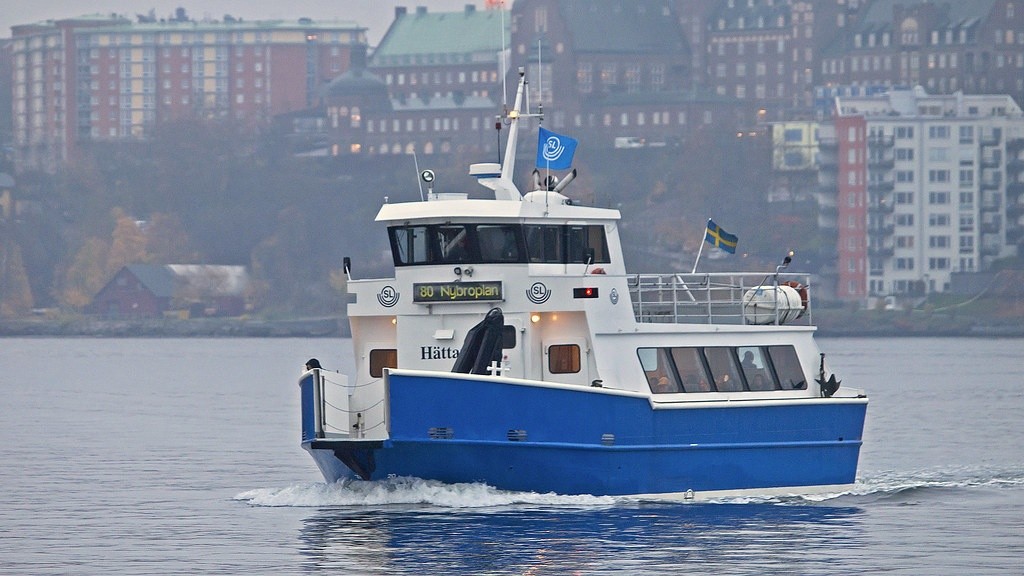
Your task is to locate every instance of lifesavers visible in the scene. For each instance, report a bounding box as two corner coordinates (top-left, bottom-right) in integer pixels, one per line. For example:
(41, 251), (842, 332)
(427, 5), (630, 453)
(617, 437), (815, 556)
(780, 280), (808, 318)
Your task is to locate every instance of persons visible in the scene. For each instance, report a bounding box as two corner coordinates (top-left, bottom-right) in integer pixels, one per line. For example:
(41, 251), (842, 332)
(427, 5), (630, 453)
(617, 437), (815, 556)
(649, 377), (676, 393)
(683, 374), (711, 392)
(741, 351), (774, 390)
(715, 372), (744, 391)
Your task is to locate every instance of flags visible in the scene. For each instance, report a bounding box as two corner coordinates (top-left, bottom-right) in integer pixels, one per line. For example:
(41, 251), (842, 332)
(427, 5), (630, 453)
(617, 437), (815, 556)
(536, 126), (578, 171)
(705, 220), (738, 254)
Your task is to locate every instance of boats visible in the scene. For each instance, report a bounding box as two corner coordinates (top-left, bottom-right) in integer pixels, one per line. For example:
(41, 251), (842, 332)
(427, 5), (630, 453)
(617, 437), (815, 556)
(298, 6), (867, 496)
(891, 313), (975, 336)
